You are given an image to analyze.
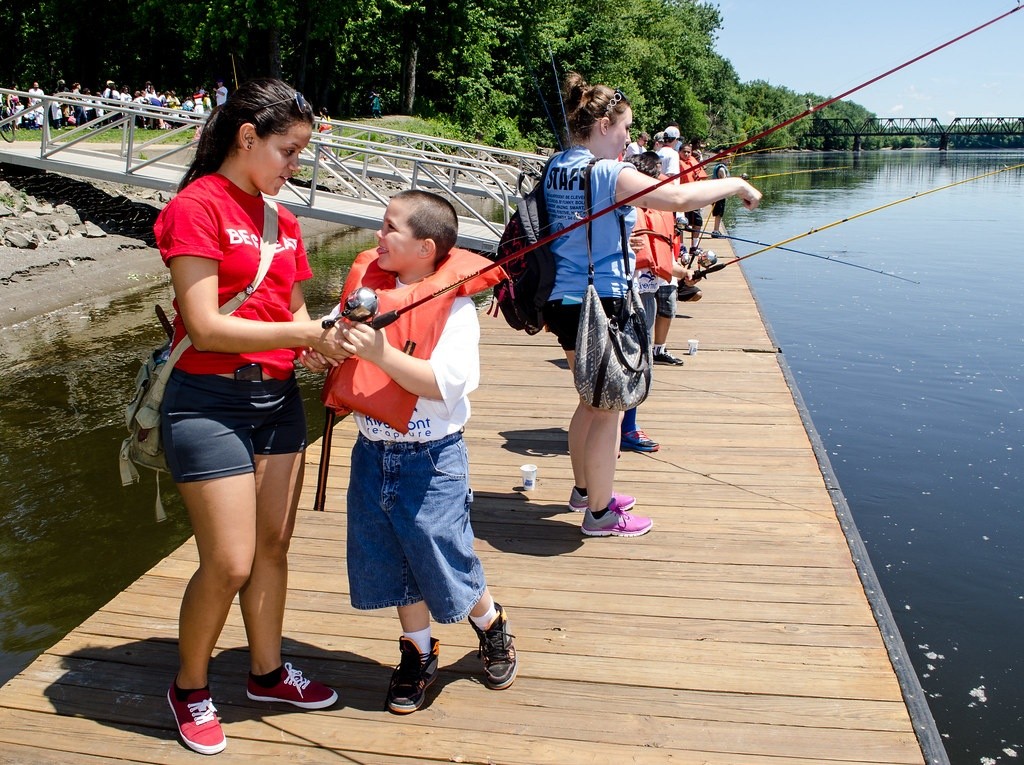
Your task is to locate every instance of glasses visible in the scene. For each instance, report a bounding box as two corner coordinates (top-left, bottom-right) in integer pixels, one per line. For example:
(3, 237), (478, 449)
(605, 89), (626, 118)
(248, 92), (313, 117)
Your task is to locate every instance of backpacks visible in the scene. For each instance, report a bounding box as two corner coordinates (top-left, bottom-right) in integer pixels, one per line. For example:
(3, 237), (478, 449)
(486, 152), (565, 336)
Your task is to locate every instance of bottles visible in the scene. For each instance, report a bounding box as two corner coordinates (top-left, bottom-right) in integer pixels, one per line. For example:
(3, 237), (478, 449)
(160, 341), (172, 365)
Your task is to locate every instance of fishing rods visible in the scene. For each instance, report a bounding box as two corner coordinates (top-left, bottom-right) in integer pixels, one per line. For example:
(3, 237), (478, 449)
(674, 224), (921, 285)
(691, 97), (853, 182)
(679, 163), (1024, 286)
(318, 1), (1024, 332)
(511, 26), (574, 152)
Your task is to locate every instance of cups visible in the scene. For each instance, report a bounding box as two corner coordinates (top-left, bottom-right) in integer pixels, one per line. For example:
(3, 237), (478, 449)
(520, 464), (537, 491)
(688, 339), (698, 355)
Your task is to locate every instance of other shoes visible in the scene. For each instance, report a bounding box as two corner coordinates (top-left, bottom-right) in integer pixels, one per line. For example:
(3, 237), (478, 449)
(689, 247), (704, 256)
(712, 231), (727, 239)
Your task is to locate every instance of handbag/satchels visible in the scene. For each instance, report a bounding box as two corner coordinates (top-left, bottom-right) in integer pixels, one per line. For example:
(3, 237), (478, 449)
(118, 343), (178, 475)
(574, 280), (651, 410)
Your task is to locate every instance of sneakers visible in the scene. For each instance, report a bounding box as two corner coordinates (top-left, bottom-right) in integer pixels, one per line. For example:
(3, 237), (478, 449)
(582, 499), (653, 537)
(468, 601), (517, 689)
(247, 662), (338, 709)
(168, 674), (227, 755)
(653, 352), (682, 365)
(621, 428), (660, 452)
(387, 637), (439, 714)
(568, 486), (636, 511)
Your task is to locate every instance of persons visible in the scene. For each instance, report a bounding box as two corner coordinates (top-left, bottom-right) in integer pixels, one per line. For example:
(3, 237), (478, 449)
(542, 72), (763, 537)
(152, 77), (341, 755)
(304, 190), (518, 713)
(0, 79), (228, 133)
(622, 125), (731, 453)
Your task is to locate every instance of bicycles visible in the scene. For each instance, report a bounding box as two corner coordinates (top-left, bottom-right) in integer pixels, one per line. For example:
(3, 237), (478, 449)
(0, 101), (16, 143)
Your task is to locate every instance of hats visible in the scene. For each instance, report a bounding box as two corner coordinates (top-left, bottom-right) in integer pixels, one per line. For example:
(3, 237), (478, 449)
(664, 126), (684, 140)
